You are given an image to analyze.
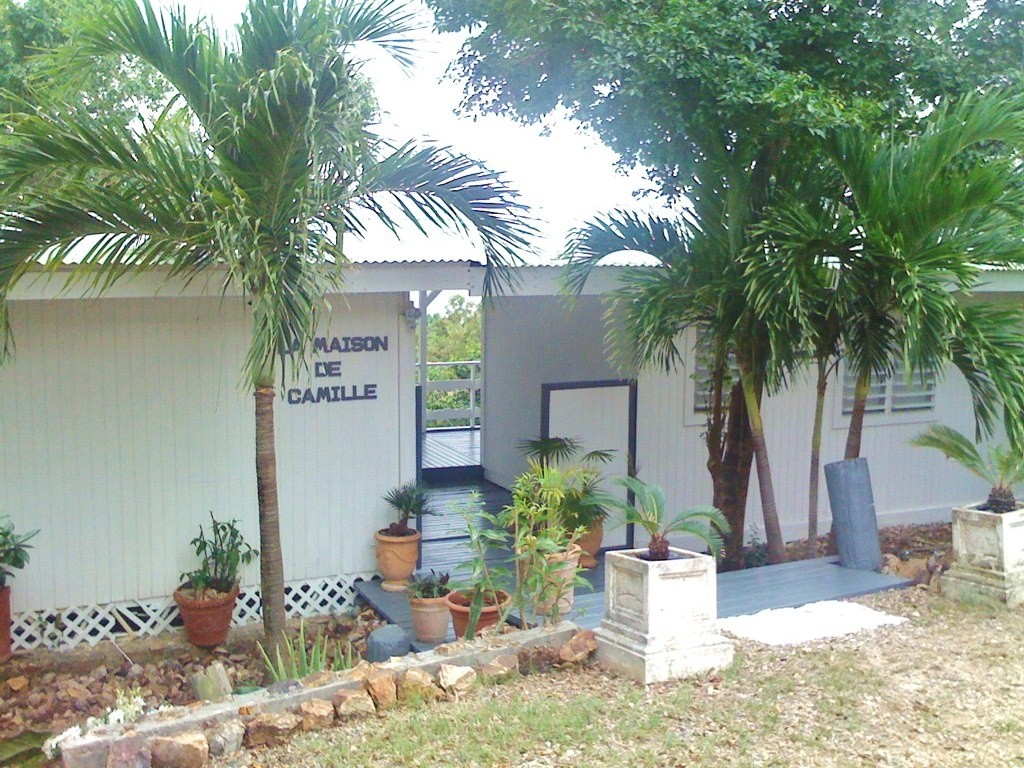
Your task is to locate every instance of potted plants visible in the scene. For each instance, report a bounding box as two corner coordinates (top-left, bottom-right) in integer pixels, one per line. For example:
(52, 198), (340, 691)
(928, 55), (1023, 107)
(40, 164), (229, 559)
(0, 512), (43, 665)
(444, 436), (614, 639)
(172, 513), (259, 649)
(373, 481), (444, 592)
(906, 422), (1024, 606)
(580, 475), (735, 687)
(403, 566), (452, 644)
